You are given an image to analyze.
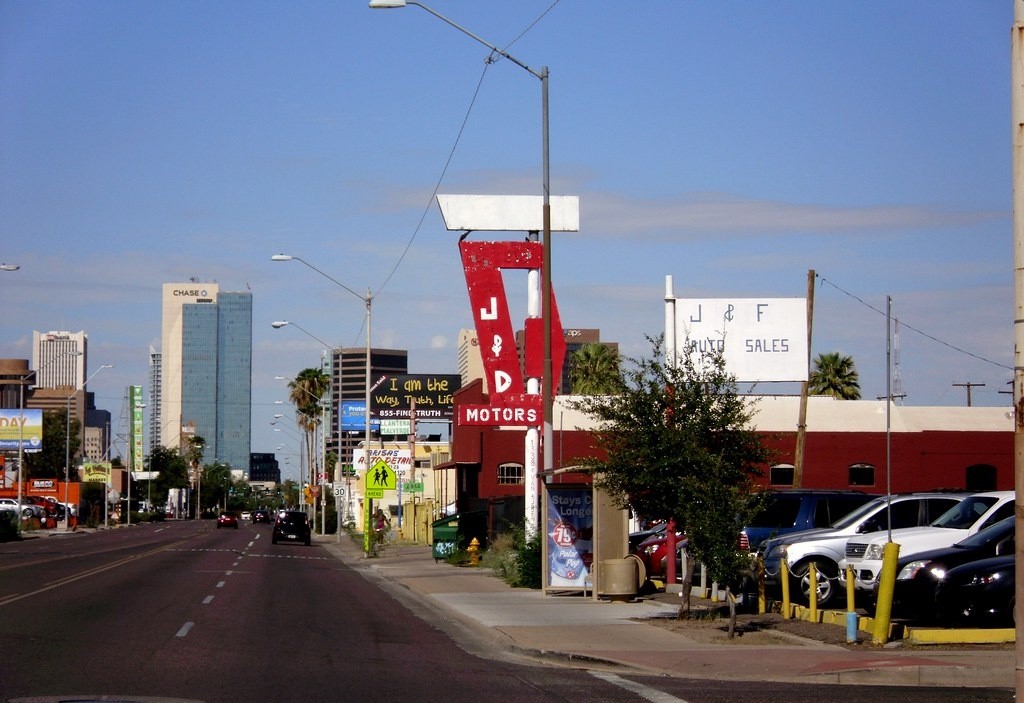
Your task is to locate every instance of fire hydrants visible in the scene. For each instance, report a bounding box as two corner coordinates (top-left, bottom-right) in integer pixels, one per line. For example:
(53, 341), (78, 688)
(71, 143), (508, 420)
(466, 536), (481, 568)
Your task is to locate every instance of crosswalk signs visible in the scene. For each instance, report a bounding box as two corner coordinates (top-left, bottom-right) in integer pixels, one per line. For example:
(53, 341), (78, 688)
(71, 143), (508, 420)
(366, 460), (396, 489)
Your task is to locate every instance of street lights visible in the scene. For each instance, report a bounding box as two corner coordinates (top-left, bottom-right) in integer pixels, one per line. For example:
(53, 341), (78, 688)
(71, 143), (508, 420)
(368, 0), (556, 482)
(126, 402), (234, 526)
(18, 350), (83, 537)
(271, 251), (374, 559)
(63, 363), (112, 531)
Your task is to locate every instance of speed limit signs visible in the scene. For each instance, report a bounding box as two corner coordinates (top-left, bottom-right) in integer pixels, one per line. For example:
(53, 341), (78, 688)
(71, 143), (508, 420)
(333, 481), (345, 497)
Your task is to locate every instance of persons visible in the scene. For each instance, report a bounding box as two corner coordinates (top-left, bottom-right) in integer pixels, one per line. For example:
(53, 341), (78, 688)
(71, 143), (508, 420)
(371, 509), (391, 546)
(71, 504), (77, 531)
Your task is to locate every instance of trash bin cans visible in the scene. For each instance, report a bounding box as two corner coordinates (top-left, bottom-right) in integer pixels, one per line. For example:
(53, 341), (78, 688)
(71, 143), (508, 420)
(431, 514), (460, 564)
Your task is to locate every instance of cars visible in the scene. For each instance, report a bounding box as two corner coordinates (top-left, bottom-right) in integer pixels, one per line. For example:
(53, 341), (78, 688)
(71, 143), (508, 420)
(241, 512), (251, 520)
(0, 495), (79, 516)
(254, 510), (270, 526)
(735, 490), (1016, 628)
(217, 510), (237, 529)
(272, 510), (314, 547)
(342, 519), (356, 528)
(629, 513), (712, 580)
